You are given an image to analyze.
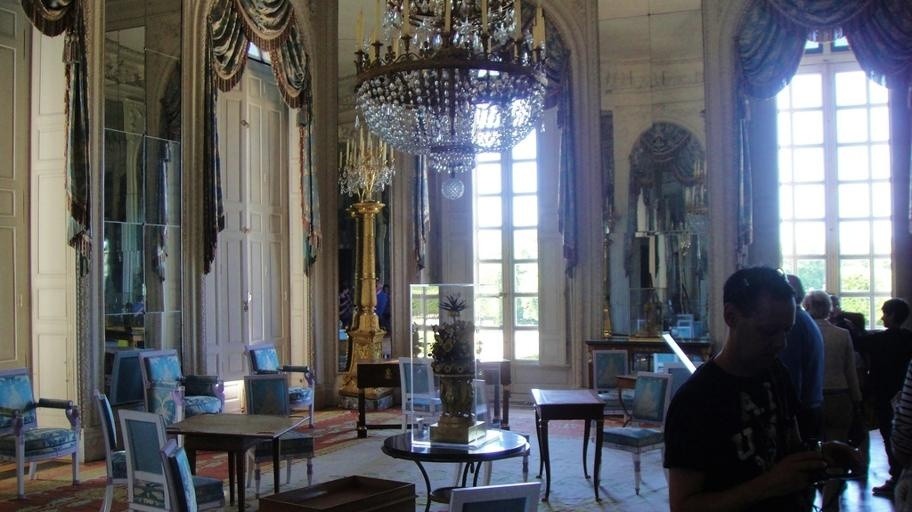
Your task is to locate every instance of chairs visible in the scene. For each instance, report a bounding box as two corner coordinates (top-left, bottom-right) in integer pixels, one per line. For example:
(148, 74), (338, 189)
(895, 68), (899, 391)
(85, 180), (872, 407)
(448, 482), (542, 512)
(398, 358), (440, 433)
(589, 369), (674, 496)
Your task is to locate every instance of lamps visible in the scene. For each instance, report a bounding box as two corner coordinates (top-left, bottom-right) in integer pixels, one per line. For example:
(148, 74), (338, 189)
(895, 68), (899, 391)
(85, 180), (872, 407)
(337, 124), (395, 401)
(352, 0), (549, 198)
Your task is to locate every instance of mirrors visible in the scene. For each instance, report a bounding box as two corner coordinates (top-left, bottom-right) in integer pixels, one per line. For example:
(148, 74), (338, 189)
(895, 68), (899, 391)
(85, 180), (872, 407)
(629, 121), (707, 327)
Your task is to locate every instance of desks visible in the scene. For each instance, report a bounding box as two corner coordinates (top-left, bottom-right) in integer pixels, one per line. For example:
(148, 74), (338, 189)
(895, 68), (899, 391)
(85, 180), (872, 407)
(531, 388), (606, 501)
(357, 355), (511, 439)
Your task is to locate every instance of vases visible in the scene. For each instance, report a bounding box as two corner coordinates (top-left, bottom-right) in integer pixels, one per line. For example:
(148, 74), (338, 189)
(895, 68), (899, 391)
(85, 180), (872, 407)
(428, 378), (486, 445)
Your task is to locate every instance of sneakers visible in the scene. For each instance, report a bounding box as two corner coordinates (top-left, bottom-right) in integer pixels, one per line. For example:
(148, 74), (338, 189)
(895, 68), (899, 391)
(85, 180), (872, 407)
(871, 479), (893, 494)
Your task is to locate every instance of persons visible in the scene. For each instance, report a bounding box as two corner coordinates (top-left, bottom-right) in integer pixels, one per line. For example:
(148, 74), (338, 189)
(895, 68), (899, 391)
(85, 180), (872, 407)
(664, 265), (908, 512)
(339, 283), (391, 335)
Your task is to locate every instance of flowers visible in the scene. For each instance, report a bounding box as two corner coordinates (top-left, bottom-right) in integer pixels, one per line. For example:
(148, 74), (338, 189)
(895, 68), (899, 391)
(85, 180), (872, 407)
(425, 294), (479, 373)
